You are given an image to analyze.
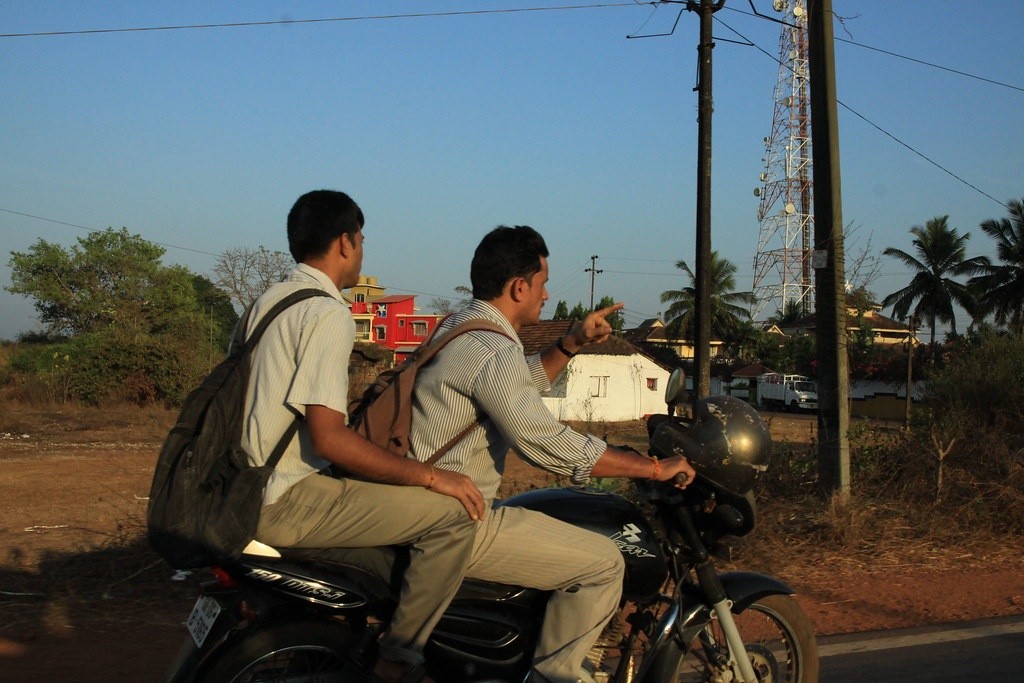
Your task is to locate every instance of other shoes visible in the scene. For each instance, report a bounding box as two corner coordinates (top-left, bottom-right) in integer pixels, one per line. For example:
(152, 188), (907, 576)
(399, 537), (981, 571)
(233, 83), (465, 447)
(523, 668), (551, 683)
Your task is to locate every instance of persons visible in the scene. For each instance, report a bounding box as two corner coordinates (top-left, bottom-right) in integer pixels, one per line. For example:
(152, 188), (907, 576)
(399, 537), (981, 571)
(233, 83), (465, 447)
(227, 189), (488, 682)
(403, 224), (695, 683)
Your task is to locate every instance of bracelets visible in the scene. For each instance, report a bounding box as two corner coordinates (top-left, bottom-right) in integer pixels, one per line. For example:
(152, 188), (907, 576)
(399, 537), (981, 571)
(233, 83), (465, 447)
(427, 463), (434, 490)
(557, 337), (578, 358)
(648, 457), (661, 480)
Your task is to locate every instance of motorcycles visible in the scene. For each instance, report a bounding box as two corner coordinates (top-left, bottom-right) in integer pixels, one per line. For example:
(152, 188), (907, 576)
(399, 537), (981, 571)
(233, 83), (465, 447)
(160, 368), (821, 683)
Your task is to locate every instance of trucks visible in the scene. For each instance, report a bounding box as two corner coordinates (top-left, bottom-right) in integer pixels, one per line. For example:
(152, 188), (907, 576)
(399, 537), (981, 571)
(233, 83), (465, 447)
(755, 371), (821, 415)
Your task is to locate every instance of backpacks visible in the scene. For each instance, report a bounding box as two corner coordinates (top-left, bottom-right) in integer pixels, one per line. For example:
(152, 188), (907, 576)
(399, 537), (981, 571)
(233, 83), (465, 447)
(146, 289), (342, 562)
(329, 311), (518, 478)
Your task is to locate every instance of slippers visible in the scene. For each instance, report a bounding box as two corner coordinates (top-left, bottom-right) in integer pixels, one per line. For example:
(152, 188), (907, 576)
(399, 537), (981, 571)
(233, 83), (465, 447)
(366, 663), (426, 683)
